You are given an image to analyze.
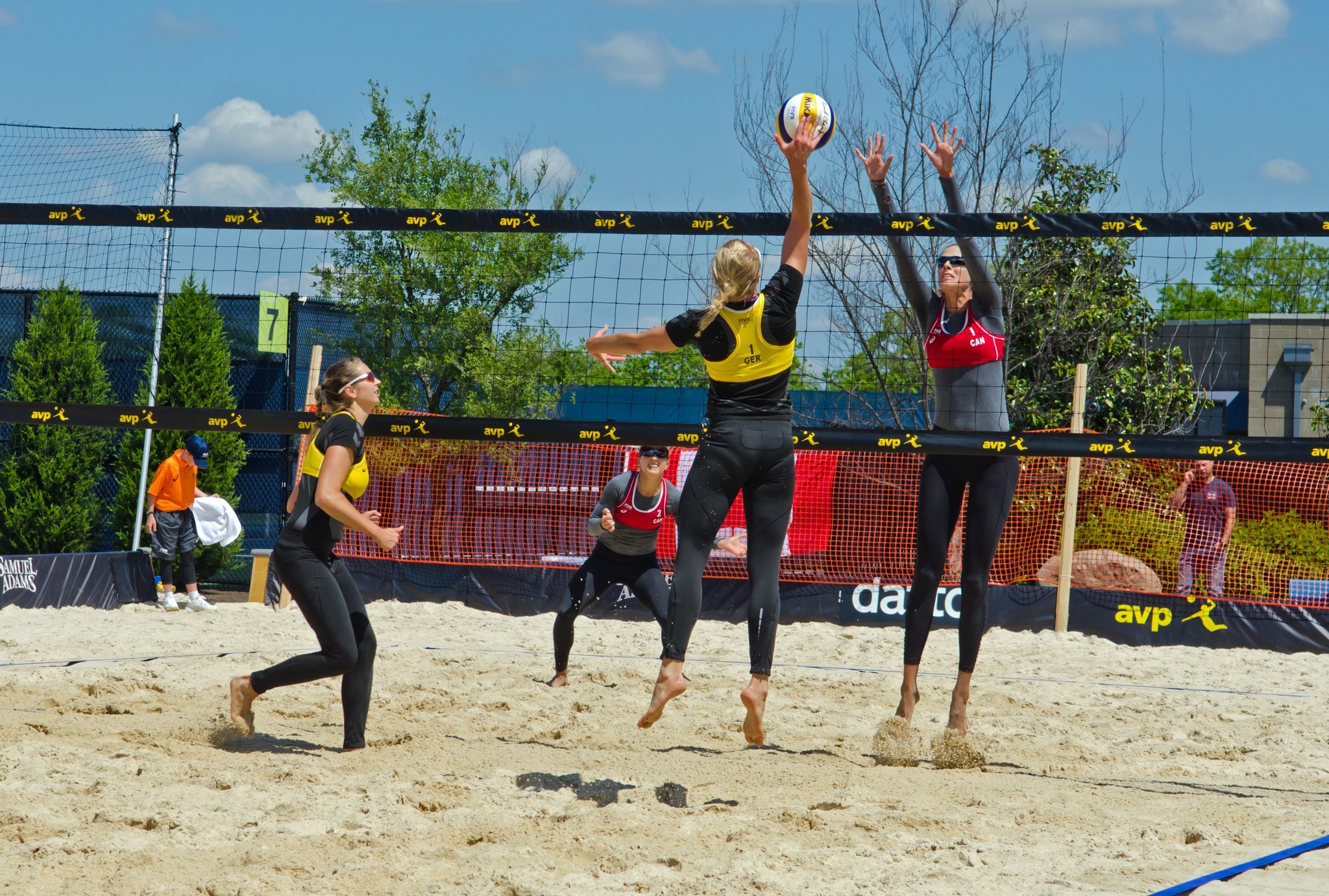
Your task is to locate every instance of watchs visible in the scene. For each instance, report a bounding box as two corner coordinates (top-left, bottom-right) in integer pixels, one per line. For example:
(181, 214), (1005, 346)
(147, 510), (154, 516)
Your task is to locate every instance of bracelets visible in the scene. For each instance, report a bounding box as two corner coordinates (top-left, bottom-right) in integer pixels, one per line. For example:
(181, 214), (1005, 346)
(1219, 538), (1229, 549)
(207, 495), (210, 498)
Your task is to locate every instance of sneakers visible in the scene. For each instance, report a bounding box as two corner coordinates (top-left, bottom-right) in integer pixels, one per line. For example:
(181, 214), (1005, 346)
(163, 595), (180, 612)
(185, 594), (218, 612)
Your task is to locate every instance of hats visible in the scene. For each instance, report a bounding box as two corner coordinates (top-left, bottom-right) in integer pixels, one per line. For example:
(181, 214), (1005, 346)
(185, 436), (209, 469)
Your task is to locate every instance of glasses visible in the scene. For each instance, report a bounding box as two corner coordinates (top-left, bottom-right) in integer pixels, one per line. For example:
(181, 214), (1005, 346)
(640, 447), (670, 459)
(936, 256), (966, 267)
(339, 371), (375, 393)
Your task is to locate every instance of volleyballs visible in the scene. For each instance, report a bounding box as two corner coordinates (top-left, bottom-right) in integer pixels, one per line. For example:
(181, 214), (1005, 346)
(775, 92), (837, 152)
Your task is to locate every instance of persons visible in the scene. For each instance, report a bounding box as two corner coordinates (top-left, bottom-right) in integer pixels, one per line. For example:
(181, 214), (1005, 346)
(586, 115), (824, 747)
(145, 437), (221, 613)
(231, 359), (404, 752)
(549, 447), (747, 694)
(1167, 460), (1235, 598)
(854, 121), (1020, 736)
